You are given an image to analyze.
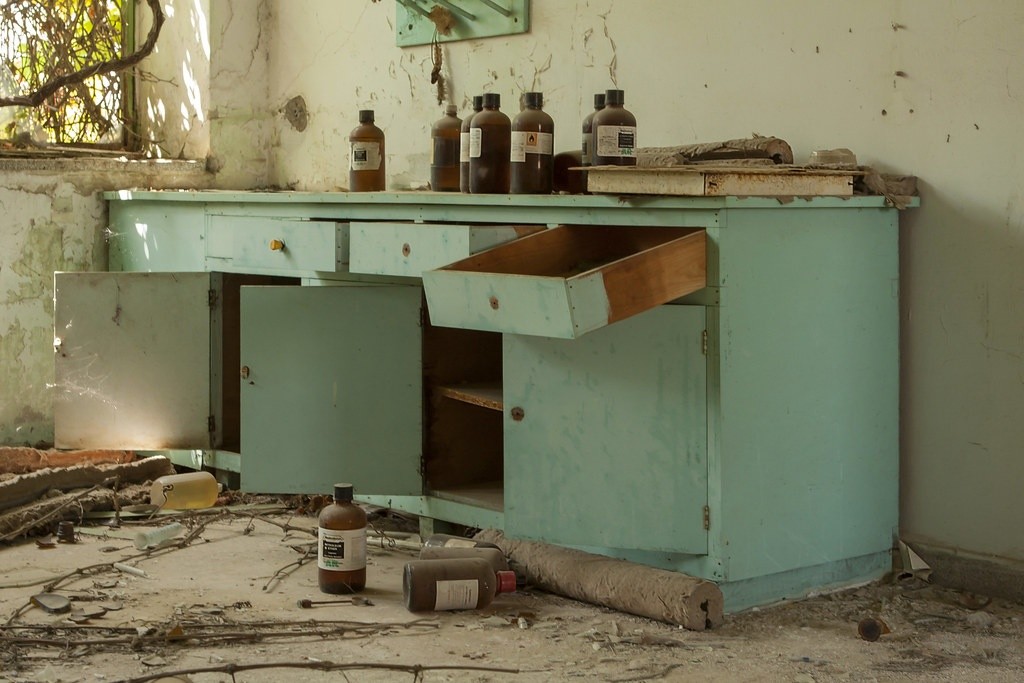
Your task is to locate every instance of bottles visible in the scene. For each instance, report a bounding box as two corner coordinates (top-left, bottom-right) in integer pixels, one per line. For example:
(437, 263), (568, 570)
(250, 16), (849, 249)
(430, 93), (637, 194)
(152, 470), (225, 509)
(317, 482), (367, 594)
(402, 535), (517, 613)
(349, 110), (385, 190)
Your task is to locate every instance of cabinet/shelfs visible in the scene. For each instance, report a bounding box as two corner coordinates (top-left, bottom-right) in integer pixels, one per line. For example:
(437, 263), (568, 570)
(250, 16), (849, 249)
(54, 189), (920, 616)
